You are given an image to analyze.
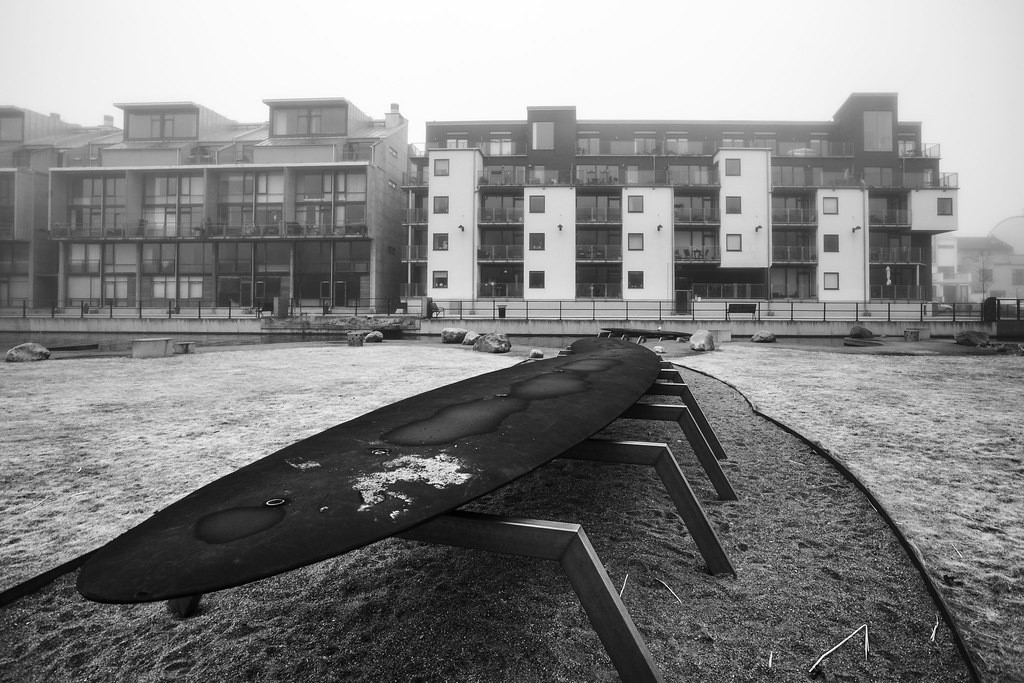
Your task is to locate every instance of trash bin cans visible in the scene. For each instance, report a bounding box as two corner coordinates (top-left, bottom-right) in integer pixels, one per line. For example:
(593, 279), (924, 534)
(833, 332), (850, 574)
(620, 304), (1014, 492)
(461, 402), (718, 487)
(497, 304), (508, 318)
(984, 297), (996, 321)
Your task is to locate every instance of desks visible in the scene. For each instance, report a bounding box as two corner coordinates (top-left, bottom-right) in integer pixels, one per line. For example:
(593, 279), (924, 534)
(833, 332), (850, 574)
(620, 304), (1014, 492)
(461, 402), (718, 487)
(692, 250), (701, 259)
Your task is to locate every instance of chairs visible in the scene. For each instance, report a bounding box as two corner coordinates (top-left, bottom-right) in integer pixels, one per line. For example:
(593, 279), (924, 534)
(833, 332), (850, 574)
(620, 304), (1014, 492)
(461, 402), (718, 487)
(698, 249), (709, 259)
(684, 249), (691, 259)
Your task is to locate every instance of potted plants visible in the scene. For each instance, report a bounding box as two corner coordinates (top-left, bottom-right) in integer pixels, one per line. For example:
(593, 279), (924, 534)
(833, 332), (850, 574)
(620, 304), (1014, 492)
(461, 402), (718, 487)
(193, 227), (202, 238)
(84, 302), (89, 314)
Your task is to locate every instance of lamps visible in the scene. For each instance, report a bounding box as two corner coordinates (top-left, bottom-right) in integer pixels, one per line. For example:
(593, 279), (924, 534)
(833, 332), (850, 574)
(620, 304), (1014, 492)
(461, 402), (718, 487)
(558, 224), (564, 231)
(458, 225), (465, 232)
(657, 225), (663, 231)
(755, 225), (763, 232)
(852, 226), (861, 233)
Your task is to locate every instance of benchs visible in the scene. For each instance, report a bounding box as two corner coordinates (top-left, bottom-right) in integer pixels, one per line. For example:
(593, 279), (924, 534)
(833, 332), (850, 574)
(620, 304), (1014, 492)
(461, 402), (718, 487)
(588, 178), (604, 185)
(107, 230), (125, 238)
(131, 338), (175, 358)
(728, 303), (757, 321)
(174, 342), (196, 355)
(431, 303), (443, 317)
